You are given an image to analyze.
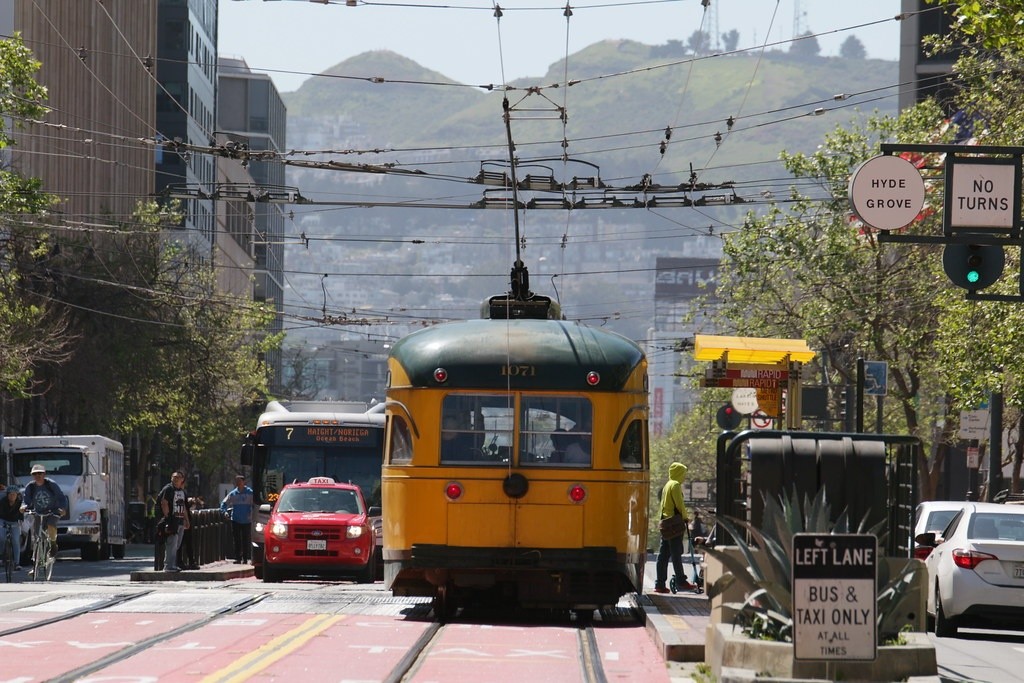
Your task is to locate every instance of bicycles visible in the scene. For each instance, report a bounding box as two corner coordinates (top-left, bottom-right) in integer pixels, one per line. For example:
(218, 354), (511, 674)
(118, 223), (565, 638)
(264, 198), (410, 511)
(22, 508), (64, 581)
(0, 521), (20, 583)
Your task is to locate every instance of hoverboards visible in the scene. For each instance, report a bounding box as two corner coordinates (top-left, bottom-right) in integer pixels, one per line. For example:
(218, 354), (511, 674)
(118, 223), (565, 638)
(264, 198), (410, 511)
(670, 520), (705, 594)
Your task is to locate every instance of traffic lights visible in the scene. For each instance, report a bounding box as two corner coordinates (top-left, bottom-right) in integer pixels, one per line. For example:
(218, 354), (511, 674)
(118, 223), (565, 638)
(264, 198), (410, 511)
(715, 404), (742, 430)
(942, 231), (1006, 291)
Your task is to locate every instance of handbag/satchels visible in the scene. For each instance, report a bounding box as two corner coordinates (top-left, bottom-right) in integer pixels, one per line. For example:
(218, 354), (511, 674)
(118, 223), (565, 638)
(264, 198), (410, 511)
(659, 514), (685, 540)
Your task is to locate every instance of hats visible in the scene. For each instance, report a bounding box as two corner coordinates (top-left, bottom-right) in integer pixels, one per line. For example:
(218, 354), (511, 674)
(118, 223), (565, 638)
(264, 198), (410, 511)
(31, 464), (45, 473)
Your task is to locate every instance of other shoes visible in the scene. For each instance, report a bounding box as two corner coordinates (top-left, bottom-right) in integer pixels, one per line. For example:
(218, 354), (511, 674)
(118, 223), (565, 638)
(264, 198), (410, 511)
(165, 565), (181, 572)
(189, 562), (200, 570)
(178, 561), (191, 570)
(233, 559), (241, 564)
(243, 559), (247, 564)
(14, 562), (22, 570)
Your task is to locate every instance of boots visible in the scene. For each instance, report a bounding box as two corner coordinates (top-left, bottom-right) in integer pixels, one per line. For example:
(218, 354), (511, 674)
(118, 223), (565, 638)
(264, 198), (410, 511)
(50, 541), (57, 557)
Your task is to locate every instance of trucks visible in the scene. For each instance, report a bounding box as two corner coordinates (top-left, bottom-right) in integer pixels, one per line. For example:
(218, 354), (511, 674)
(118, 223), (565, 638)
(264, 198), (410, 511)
(2, 434), (125, 562)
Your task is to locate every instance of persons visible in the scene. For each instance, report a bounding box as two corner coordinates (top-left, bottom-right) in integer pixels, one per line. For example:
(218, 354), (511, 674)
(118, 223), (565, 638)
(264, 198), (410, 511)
(0, 486), (23, 571)
(19, 464), (68, 575)
(221, 476), (253, 564)
(686, 511), (707, 554)
(705, 525), (717, 549)
(155, 471), (200, 572)
(654, 461), (697, 593)
(0, 482), (7, 499)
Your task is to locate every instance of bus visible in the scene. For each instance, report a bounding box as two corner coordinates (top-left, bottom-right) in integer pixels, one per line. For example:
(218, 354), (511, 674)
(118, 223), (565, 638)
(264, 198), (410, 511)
(238, 398), (386, 580)
(379, 93), (652, 620)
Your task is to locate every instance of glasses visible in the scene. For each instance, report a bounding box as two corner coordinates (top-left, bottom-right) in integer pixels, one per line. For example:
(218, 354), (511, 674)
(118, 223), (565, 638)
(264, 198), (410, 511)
(32, 473), (44, 475)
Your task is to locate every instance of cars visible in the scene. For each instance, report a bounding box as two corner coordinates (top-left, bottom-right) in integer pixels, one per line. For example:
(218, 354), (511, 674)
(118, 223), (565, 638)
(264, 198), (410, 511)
(905, 500), (1024, 638)
(258, 476), (376, 584)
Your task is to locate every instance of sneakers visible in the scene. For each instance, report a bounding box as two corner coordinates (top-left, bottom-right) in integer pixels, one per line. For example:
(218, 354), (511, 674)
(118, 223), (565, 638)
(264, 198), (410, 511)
(677, 581), (696, 591)
(655, 585), (670, 593)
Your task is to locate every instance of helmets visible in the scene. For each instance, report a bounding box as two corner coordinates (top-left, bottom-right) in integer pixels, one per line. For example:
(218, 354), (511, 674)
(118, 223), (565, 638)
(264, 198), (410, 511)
(7, 485), (19, 494)
